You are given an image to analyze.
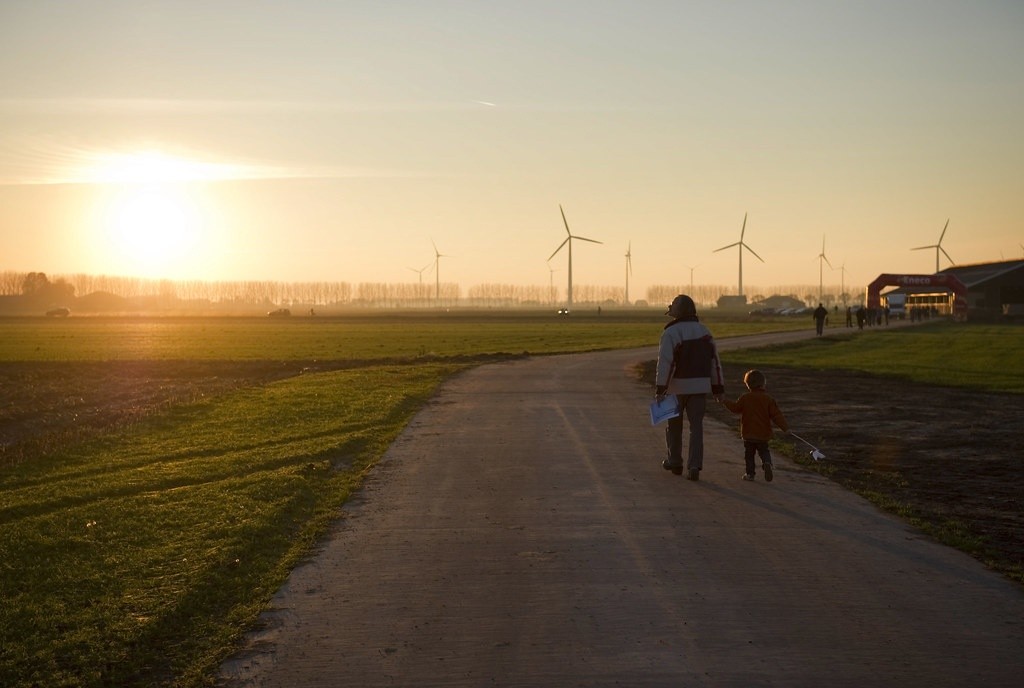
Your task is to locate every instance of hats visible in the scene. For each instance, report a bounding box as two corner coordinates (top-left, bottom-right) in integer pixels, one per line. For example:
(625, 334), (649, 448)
(664, 294), (696, 319)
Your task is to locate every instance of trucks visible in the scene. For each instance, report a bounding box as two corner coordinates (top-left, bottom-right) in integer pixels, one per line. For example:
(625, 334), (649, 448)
(886, 293), (907, 321)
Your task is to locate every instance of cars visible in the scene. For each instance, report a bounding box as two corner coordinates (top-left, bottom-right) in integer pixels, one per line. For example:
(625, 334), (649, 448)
(748, 306), (816, 317)
(267, 308), (290, 316)
(46, 307), (71, 317)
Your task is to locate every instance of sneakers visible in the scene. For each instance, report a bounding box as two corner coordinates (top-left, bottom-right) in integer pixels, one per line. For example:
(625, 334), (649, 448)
(741, 473), (755, 481)
(764, 462), (774, 482)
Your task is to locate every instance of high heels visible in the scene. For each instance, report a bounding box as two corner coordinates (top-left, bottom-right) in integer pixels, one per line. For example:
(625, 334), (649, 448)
(684, 466), (699, 481)
(662, 459), (683, 475)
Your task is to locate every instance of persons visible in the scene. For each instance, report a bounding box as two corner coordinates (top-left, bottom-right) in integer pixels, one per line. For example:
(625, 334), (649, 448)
(655, 294), (724, 480)
(724, 369), (792, 482)
(814, 303), (829, 335)
(910, 306), (940, 323)
(846, 305), (890, 330)
